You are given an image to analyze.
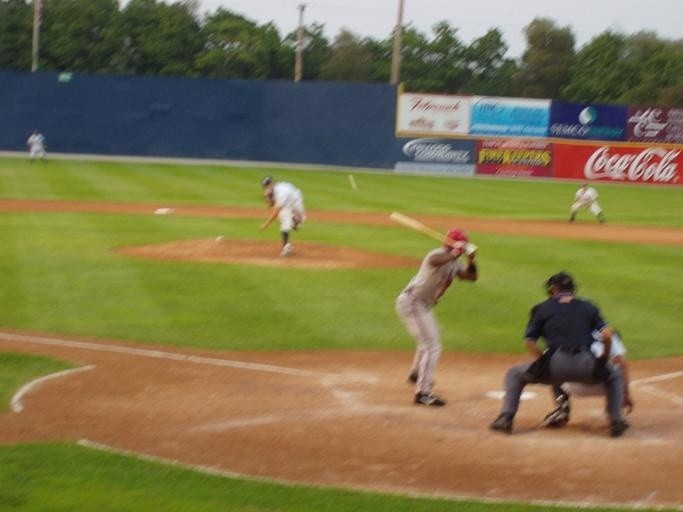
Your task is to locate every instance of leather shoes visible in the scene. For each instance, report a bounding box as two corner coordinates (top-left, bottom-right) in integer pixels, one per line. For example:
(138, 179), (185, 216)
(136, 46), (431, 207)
(490, 414), (513, 434)
(613, 424), (628, 435)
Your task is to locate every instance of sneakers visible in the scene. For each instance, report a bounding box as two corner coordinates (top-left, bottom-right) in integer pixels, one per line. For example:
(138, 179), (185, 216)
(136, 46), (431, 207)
(544, 406), (570, 426)
(409, 373), (445, 406)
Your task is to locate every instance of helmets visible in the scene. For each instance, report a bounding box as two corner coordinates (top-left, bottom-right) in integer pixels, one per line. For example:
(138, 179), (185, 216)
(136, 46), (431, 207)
(443, 230), (468, 254)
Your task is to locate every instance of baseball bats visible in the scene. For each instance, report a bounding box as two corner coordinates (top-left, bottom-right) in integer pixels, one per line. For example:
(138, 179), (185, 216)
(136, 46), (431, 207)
(390, 210), (478, 255)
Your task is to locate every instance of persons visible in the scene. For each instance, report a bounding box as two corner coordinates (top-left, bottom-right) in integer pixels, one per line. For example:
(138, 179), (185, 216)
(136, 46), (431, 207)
(26, 128), (49, 167)
(395, 228), (478, 407)
(489, 270), (633, 436)
(259, 176), (306, 257)
(568, 183), (607, 223)
